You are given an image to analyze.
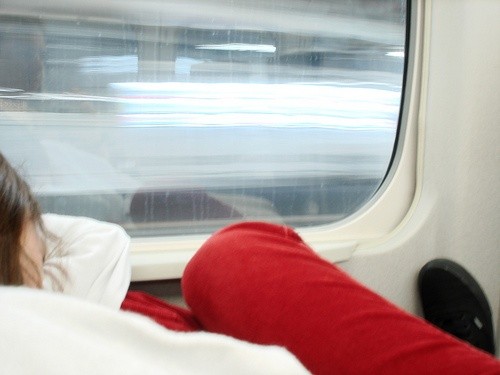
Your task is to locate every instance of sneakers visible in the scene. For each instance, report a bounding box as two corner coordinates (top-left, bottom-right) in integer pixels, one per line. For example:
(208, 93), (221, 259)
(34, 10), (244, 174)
(418, 258), (495, 359)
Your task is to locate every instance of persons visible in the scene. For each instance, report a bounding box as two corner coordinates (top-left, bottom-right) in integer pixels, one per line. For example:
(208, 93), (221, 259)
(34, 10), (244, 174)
(0, 154), (500, 374)
(0, 15), (53, 131)
